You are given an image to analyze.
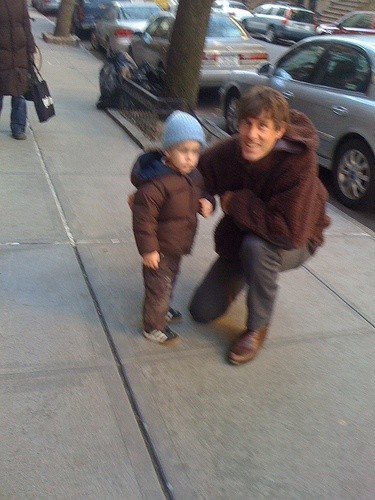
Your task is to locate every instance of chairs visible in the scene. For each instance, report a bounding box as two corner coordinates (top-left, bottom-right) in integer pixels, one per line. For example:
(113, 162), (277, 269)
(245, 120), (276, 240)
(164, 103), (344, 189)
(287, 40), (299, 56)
(331, 60), (356, 86)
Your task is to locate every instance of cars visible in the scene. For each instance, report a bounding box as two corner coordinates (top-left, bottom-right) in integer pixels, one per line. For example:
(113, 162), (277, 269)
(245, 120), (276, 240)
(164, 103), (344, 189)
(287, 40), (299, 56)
(120, 12), (270, 95)
(315, 10), (375, 59)
(249, 2), (322, 45)
(220, 34), (375, 211)
(211, 0), (256, 24)
(31, 0), (180, 62)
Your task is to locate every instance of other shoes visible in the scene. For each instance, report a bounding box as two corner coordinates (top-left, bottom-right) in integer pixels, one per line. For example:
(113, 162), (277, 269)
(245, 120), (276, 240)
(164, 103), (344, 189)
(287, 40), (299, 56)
(12, 132), (26, 140)
(142, 323), (182, 347)
(165, 306), (183, 323)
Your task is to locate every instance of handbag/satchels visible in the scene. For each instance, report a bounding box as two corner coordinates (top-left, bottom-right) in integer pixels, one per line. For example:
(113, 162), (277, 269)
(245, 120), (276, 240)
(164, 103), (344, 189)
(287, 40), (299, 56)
(24, 66), (38, 101)
(29, 60), (56, 122)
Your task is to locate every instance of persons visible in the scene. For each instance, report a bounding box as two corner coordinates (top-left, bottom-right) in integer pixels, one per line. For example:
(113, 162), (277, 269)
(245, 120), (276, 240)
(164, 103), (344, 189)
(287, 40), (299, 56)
(0, 0), (36, 140)
(132, 111), (216, 347)
(129, 86), (330, 364)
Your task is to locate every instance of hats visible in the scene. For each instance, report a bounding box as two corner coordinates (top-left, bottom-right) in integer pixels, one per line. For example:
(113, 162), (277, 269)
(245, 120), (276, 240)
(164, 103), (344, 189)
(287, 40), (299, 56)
(161, 109), (207, 154)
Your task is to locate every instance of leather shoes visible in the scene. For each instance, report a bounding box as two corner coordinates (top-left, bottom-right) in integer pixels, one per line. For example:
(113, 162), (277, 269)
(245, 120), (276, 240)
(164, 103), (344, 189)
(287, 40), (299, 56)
(226, 322), (268, 365)
